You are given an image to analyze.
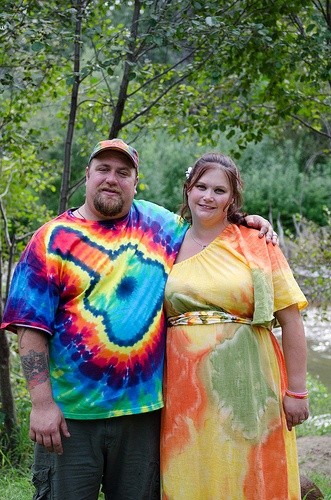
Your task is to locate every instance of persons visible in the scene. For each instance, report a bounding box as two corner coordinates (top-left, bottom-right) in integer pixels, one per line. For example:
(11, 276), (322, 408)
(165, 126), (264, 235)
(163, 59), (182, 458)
(160, 153), (309, 499)
(0, 139), (279, 500)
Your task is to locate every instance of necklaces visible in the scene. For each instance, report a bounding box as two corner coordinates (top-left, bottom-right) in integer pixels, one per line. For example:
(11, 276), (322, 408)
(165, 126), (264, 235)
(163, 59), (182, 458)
(76, 209), (85, 219)
(190, 228), (207, 248)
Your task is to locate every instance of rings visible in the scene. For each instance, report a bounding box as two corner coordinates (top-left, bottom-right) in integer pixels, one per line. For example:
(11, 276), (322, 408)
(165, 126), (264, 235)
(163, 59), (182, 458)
(299, 420), (304, 423)
(273, 234), (278, 238)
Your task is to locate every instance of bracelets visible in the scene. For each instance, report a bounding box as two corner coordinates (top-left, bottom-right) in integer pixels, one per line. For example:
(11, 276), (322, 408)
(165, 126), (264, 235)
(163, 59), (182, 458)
(286, 389), (308, 399)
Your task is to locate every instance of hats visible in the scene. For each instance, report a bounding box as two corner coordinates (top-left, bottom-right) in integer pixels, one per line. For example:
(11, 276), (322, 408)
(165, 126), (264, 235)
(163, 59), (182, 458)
(88, 139), (139, 168)
